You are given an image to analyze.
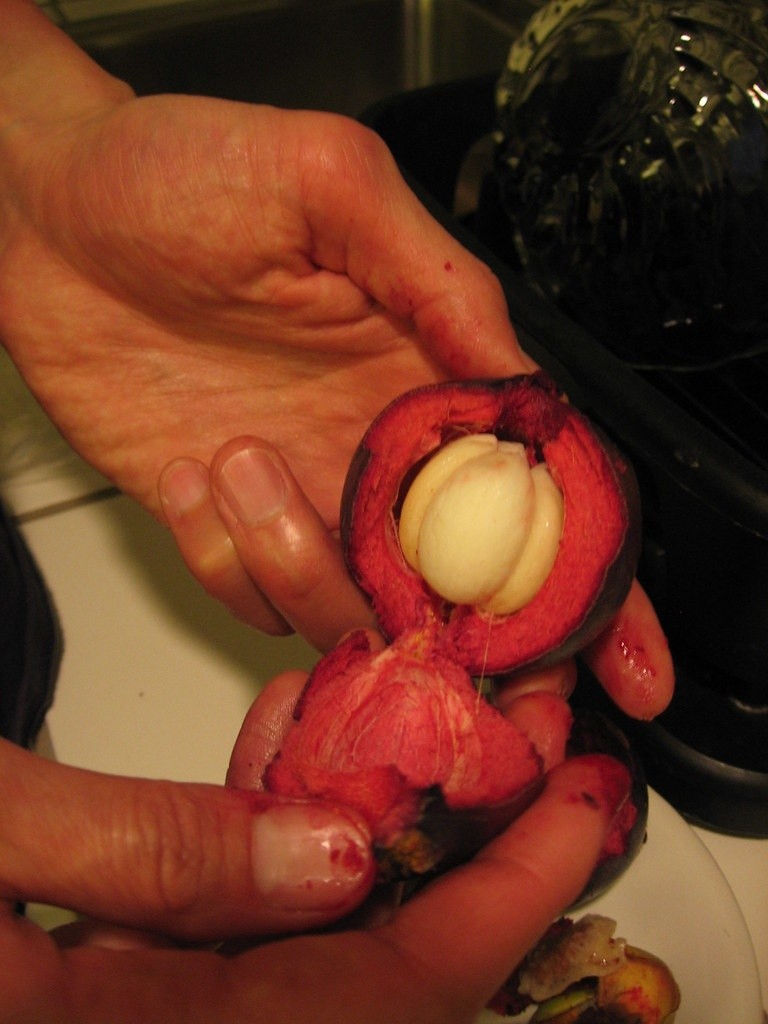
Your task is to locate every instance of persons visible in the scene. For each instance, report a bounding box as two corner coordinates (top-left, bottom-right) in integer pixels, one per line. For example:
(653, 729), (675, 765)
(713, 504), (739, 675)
(3, 0), (676, 1024)
